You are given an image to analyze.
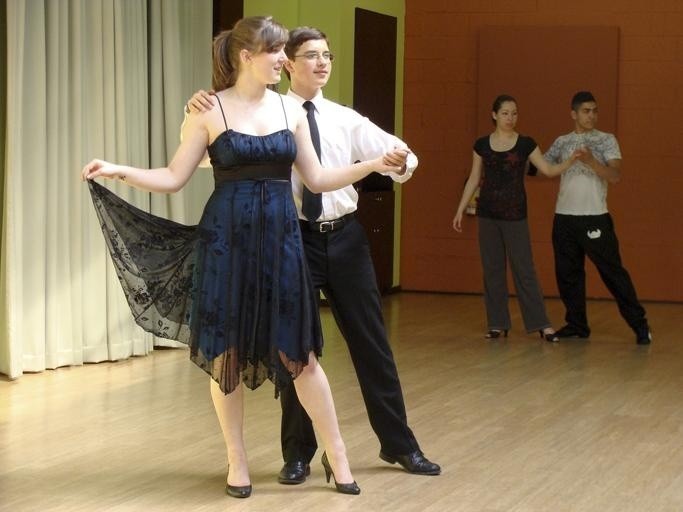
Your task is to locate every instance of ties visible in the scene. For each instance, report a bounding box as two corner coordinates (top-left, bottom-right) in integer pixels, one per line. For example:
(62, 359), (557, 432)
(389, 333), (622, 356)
(301, 100), (324, 225)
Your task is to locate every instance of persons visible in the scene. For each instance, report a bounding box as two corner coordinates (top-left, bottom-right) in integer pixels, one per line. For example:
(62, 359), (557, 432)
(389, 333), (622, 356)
(180, 26), (439, 484)
(451, 95), (581, 341)
(82, 13), (409, 499)
(525, 92), (654, 345)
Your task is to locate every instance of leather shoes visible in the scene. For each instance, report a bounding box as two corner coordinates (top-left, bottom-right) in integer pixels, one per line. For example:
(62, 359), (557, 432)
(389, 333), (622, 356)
(277, 458), (312, 486)
(378, 447), (444, 477)
(636, 328), (653, 346)
(555, 324), (592, 339)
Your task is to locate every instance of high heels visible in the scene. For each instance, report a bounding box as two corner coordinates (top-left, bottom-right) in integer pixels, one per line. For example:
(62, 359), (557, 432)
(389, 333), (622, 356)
(226, 461), (253, 500)
(484, 328), (509, 340)
(320, 449), (362, 496)
(539, 326), (560, 342)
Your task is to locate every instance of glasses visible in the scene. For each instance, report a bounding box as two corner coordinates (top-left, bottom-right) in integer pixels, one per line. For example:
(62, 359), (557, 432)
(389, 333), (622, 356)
(288, 52), (335, 61)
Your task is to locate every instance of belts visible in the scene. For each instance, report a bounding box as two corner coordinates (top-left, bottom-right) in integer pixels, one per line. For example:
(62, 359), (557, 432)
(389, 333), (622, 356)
(297, 208), (361, 236)
